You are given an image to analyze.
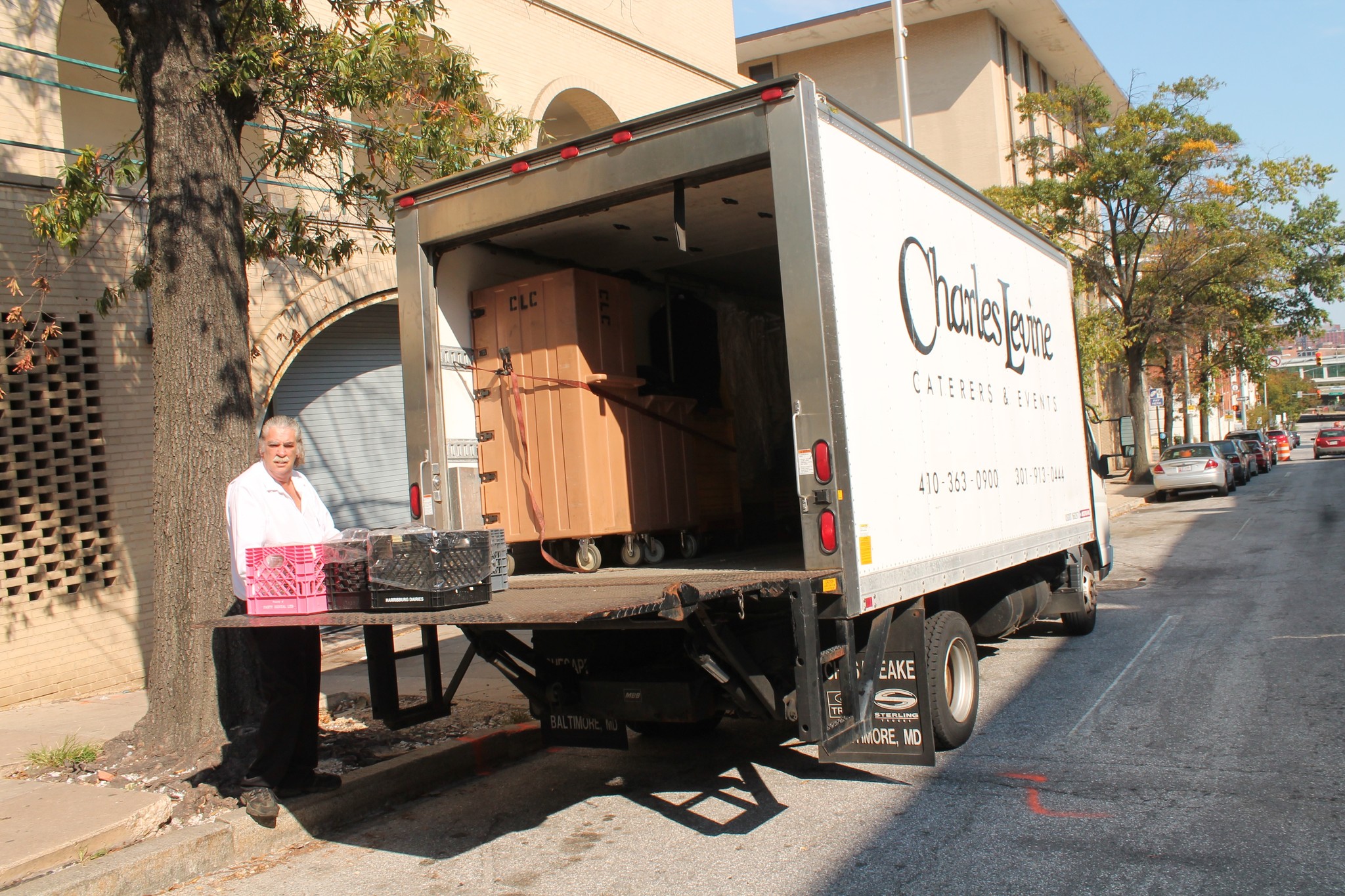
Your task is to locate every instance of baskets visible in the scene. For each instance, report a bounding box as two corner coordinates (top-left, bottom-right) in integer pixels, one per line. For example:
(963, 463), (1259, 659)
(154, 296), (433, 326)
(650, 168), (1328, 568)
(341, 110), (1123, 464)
(246, 528), (510, 617)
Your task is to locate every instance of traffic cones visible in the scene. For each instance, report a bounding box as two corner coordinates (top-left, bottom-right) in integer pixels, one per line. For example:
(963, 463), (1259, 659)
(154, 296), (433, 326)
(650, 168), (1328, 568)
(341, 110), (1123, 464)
(1277, 443), (1291, 462)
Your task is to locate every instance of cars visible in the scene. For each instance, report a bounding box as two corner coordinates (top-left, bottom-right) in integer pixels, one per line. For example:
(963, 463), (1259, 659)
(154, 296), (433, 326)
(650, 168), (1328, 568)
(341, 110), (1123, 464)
(1265, 435), (1277, 465)
(1265, 430), (1300, 450)
(1153, 439), (1273, 500)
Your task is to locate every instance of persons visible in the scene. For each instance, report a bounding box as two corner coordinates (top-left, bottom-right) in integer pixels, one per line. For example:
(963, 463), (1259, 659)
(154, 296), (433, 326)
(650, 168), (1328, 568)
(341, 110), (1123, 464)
(224, 415), (343, 797)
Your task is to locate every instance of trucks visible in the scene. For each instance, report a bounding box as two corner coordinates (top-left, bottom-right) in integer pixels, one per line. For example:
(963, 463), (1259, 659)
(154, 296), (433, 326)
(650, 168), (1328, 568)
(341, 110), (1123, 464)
(391, 72), (1136, 760)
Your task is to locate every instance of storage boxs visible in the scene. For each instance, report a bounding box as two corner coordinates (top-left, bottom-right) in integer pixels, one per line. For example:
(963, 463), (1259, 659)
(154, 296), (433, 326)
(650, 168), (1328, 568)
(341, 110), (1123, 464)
(461, 263), (794, 575)
(362, 528), (494, 613)
(245, 539), (374, 618)
(487, 529), (511, 592)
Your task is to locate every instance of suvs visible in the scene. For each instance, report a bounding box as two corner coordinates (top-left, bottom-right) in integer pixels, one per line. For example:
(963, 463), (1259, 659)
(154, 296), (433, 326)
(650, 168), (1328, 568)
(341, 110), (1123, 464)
(1311, 426), (1345, 459)
(1225, 429), (1273, 470)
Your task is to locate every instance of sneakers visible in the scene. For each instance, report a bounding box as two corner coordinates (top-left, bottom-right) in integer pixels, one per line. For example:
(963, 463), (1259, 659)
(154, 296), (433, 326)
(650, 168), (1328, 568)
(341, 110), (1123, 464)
(240, 788), (280, 818)
(276, 772), (342, 798)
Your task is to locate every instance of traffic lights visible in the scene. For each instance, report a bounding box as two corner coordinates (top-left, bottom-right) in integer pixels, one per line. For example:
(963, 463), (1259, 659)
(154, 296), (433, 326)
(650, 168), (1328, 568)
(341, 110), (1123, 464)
(1315, 352), (1322, 367)
(1233, 405), (1239, 411)
(1316, 390), (1321, 399)
